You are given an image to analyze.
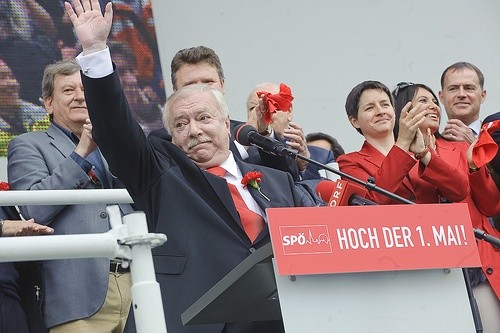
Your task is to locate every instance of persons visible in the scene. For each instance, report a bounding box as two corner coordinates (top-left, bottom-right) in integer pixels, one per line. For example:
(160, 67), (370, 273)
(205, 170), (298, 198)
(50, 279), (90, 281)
(336, 81), (470, 205)
(246, 82), (334, 180)
(393, 62), (500, 333)
(7, 60), (133, 333)
(0, 0), (164, 155)
(65, 0), (302, 333)
(149, 46), (298, 176)
(0, 207), (55, 333)
(306, 132), (345, 161)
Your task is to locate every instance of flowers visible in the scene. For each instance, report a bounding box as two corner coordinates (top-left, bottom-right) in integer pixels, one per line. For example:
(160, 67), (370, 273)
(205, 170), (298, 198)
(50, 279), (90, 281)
(0, 181), (9, 191)
(241, 171), (270, 201)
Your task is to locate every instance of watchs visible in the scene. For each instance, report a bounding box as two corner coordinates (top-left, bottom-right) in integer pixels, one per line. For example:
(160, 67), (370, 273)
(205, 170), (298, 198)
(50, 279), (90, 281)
(259, 125), (272, 136)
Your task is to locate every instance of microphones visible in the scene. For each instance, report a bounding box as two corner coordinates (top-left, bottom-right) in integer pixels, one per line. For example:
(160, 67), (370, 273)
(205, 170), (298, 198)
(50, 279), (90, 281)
(233, 123), (288, 157)
(316, 181), (381, 206)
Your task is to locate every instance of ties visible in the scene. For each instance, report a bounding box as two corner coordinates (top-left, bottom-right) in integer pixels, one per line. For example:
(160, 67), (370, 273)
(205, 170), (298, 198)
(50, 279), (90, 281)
(206, 167), (266, 243)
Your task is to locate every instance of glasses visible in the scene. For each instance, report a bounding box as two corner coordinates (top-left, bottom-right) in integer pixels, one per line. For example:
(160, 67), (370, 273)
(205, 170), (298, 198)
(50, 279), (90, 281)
(395, 82), (418, 96)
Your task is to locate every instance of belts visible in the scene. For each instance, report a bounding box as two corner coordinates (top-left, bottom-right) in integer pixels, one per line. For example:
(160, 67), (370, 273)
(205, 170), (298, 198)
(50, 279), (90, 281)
(110, 261), (130, 272)
